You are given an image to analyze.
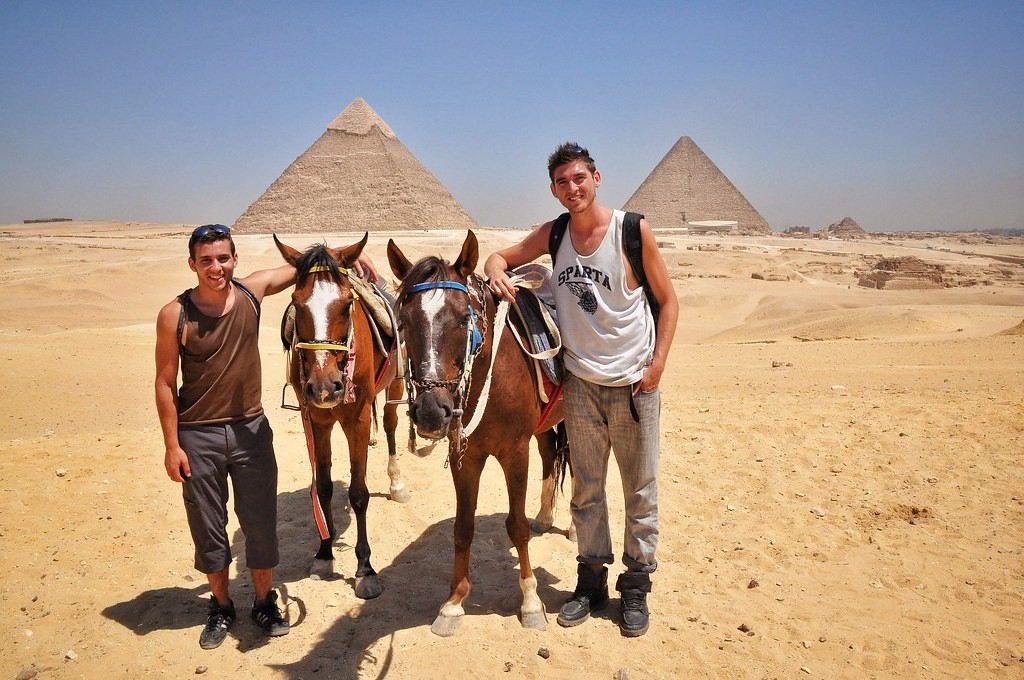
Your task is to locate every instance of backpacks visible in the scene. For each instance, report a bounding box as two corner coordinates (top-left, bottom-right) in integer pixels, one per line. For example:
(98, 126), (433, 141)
(548, 210), (658, 330)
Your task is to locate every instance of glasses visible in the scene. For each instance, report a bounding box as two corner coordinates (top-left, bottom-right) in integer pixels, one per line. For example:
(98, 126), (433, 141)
(195, 225), (229, 236)
(567, 146), (582, 153)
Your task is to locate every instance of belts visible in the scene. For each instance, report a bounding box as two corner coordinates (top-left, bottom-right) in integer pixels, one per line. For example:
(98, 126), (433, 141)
(628, 384), (640, 423)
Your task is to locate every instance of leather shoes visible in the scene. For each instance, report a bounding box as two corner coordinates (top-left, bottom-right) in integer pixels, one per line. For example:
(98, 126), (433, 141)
(557, 562), (610, 627)
(616, 571), (653, 637)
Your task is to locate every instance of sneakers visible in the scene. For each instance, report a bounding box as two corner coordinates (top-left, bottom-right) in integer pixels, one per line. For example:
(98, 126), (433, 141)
(199, 596), (237, 649)
(250, 590), (290, 636)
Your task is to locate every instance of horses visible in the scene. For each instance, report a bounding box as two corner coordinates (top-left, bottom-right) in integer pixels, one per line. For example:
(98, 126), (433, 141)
(271, 232), (573, 637)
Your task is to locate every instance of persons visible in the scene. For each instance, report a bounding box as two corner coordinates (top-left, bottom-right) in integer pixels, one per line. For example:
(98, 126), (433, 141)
(484, 140), (679, 636)
(155, 224), (378, 649)
